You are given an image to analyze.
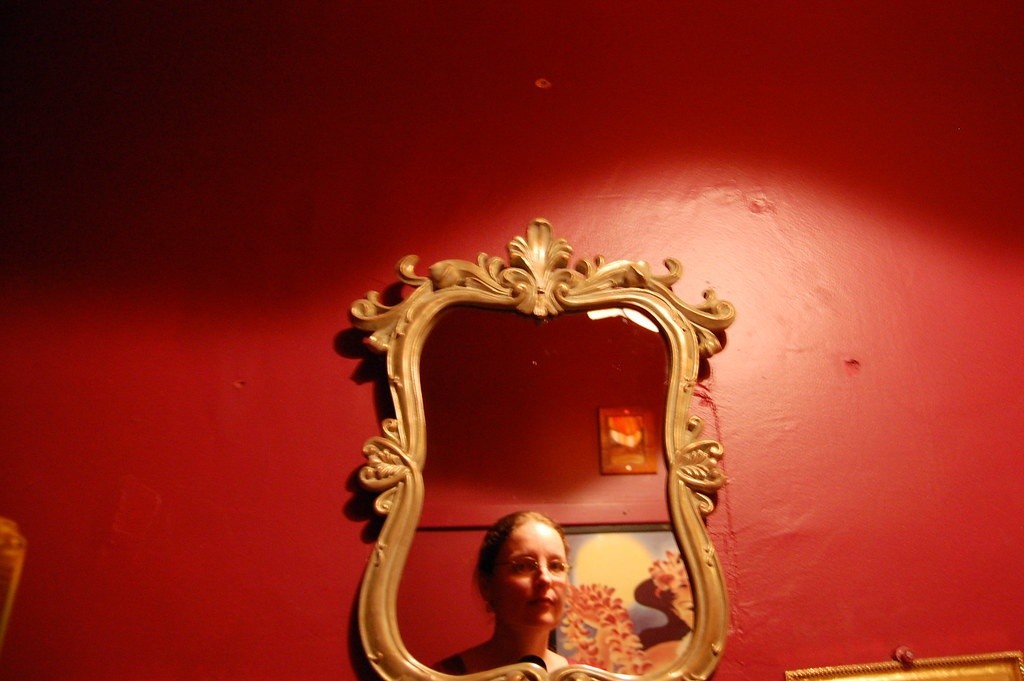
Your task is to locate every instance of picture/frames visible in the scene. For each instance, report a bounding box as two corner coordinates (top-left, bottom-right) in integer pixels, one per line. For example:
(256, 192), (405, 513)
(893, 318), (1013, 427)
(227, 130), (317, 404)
(598, 406), (659, 474)
(547, 519), (695, 675)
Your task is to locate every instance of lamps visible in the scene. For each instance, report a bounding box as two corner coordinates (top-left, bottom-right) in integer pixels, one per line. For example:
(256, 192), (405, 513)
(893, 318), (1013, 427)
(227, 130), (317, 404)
(347, 219), (737, 681)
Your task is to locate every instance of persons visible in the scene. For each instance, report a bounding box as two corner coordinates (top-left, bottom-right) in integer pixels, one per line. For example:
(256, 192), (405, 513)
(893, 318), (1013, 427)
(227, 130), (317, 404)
(431, 511), (607, 673)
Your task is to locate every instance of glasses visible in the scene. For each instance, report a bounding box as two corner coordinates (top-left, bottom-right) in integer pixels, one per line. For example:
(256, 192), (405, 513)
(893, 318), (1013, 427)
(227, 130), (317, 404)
(495, 556), (570, 575)
